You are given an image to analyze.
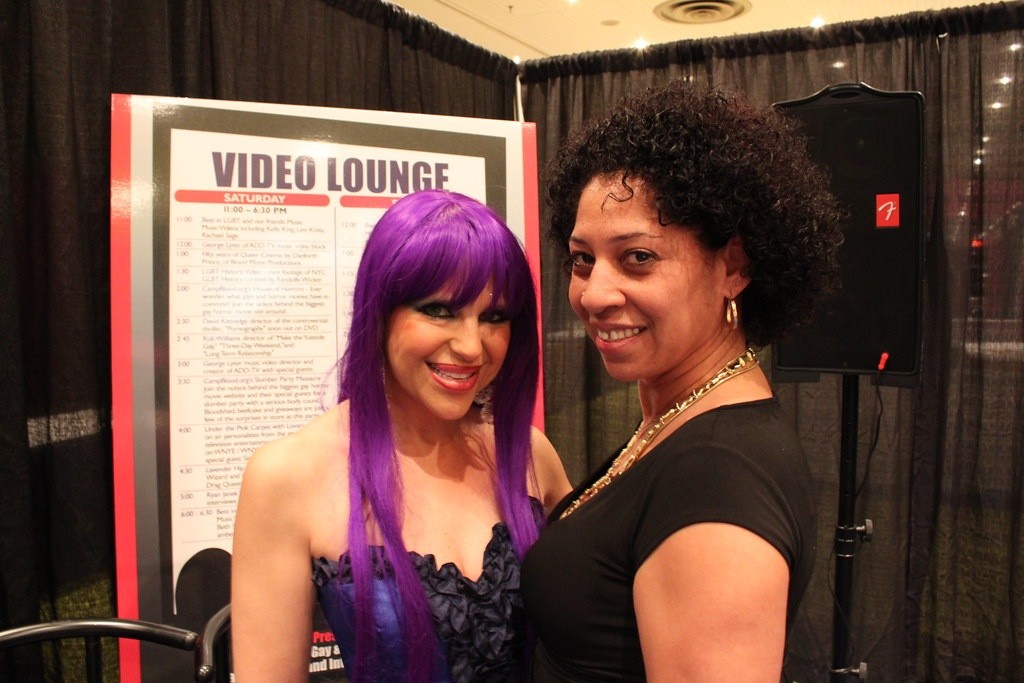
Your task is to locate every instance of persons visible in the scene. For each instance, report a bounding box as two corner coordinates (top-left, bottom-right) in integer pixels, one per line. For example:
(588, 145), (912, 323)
(517, 80), (844, 683)
(229, 189), (573, 683)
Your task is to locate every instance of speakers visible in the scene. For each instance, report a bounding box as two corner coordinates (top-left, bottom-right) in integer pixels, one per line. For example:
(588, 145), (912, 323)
(769, 82), (925, 376)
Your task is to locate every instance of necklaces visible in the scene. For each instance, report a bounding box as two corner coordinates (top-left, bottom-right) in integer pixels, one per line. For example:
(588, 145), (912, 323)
(564, 345), (758, 517)
